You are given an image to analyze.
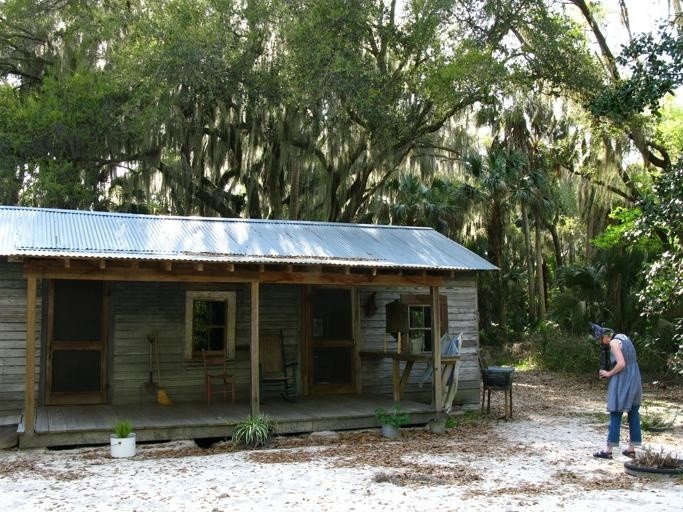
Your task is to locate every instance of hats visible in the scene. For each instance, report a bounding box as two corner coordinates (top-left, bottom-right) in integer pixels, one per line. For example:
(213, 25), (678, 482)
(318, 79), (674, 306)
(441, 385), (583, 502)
(592, 324), (613, 340)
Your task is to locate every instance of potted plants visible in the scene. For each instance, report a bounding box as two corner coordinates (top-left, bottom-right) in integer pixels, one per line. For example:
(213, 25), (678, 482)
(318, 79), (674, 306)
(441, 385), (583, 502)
(373, 402), (411, 437)
(226, 412), (281, 452)
(111, 417), (137, 457)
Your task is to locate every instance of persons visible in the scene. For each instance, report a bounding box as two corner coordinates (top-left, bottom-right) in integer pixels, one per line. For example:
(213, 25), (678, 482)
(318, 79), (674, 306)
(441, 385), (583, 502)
(589, 322), (642, 459)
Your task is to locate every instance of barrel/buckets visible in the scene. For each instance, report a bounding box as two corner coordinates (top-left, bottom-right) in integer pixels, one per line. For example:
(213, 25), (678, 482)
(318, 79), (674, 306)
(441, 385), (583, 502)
(111, 434), (135, 457)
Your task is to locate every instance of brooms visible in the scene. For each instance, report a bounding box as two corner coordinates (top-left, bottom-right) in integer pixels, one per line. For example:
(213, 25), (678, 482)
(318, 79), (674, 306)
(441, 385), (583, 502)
(154, 334), (173, 405)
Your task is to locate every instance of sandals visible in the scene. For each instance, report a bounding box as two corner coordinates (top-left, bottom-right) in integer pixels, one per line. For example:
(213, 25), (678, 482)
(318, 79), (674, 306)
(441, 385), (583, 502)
(593, 449), (614, 460)
(622, 449), (636, 456)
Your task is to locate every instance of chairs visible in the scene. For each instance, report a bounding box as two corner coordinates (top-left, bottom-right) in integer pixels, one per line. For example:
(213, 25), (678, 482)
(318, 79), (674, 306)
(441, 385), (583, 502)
(202, 348), (236, 407)
(260, 328), (299, 404)
(477, 356), (512, 421)
